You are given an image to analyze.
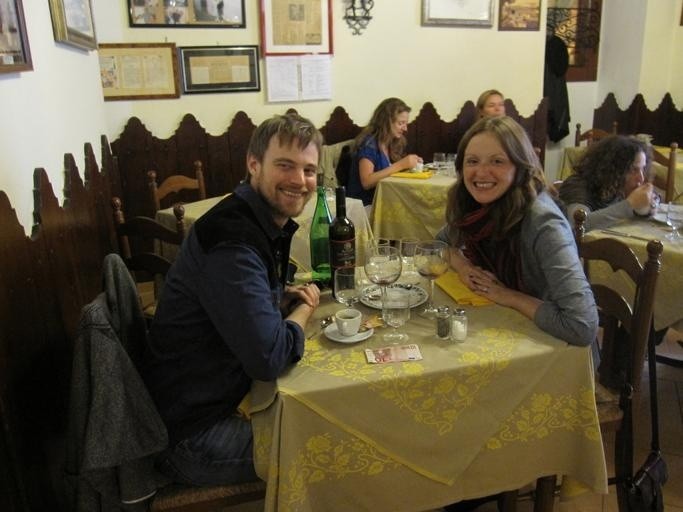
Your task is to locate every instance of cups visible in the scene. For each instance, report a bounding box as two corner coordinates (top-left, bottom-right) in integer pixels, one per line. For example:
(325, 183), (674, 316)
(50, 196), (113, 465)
(333, 310), (362, 337)
(331, 266), (362, 306)
(364, 237), (391, 261)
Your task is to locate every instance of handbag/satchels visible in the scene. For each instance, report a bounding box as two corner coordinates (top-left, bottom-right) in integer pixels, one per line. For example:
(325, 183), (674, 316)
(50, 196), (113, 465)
(624, 453), (668, 512)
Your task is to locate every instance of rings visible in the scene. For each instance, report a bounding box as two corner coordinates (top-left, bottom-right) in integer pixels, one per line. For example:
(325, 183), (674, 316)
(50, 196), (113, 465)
(482, 288), (488, 292)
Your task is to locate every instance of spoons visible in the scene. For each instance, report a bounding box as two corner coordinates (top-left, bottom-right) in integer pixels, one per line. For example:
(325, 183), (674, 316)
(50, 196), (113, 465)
(305, 316), (331, 341)
(405, 282), (423, 289)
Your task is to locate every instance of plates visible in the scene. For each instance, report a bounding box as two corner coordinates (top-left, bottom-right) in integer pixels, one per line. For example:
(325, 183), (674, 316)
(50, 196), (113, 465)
(424, 161), (452, 169)
(360, 283), (428, 310)
(650, 203), (683, 224)
(323, 323), (373, 344)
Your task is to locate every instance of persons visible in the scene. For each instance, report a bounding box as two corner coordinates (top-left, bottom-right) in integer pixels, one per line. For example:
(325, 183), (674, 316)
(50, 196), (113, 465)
(348, 97), (424, 245)
(476, 90), (506, 121)
(135, 113), (323, 488)
(435, 114), (601, 368)
(563, 134), (660, 237)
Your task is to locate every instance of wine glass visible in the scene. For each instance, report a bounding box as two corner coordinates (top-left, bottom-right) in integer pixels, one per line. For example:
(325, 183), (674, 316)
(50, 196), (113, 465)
(401, 240), (417, 278)
(379, 293), (409, 346)
(363, 248), (399, 301)
(414, 240), (452, 321)
(662, 200), (682, 242)
(432, 152), (458, 178)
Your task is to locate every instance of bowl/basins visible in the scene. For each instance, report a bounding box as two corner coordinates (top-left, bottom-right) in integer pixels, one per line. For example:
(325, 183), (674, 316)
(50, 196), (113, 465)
(633, 196), (658, 216)
(409, 163), (423, 173)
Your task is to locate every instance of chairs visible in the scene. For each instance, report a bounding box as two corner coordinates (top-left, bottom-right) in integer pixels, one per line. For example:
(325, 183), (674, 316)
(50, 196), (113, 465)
(319, 139), (358, 192)
(498, 209), (663, 511)
(110, 197), (185, 333)
(644, 142), (678, 204)
(576, 121), (618, 147)
(53, 253), (266, 512)
(147, 160), (206, 210)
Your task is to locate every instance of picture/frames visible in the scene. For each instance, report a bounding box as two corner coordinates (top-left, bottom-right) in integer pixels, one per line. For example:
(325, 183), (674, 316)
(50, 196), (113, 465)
(128, 0), (246, 28)
(48, 0), (97, 50)
(421, 0), (494, 28)
(498, 0), (541, 31)
(259, 0), (333, 55)
(0, 0), (33, 73)
(177, 45), (260, 94)
(97, 43), (180, 101)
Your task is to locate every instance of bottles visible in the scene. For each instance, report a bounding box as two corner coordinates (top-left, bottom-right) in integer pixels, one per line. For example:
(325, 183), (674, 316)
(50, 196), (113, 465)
(450, 309), (467, 343)
(434, 304), (452, 339)
(327, 187), (357, 295)
(308, 185), (334, 286)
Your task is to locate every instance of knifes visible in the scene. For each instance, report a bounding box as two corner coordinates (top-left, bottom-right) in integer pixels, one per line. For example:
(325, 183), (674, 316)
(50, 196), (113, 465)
(599, 229), (650, 242)
